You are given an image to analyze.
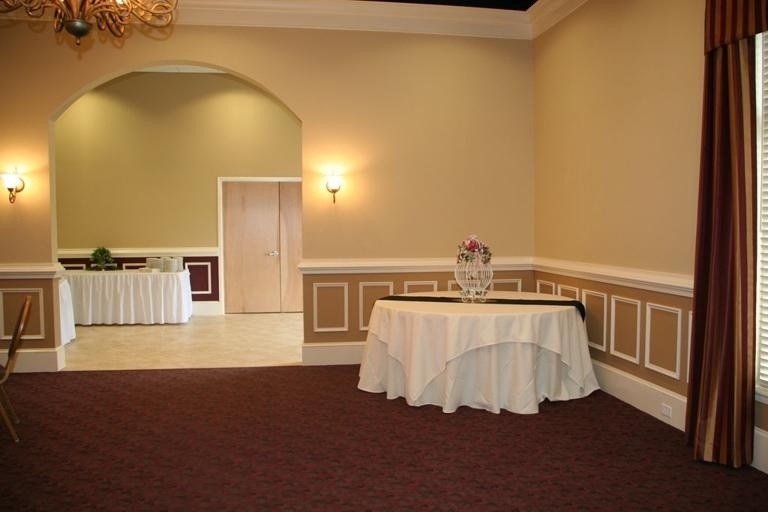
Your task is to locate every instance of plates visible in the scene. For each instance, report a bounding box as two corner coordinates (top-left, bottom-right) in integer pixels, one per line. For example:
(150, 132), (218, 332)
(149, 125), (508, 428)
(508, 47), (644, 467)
(139, 256), (184, 272)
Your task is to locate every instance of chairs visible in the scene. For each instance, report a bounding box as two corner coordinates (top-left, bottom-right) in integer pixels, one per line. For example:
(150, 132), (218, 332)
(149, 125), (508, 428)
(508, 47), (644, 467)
(0, 294), (30, 444)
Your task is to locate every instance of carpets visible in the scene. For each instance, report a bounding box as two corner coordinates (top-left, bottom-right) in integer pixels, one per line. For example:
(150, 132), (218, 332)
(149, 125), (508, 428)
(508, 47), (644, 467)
(0, 363), (768, 512)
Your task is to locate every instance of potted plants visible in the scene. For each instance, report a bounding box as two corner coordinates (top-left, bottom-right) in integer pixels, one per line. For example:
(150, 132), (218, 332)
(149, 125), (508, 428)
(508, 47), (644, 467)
(90, 247), (112, 271)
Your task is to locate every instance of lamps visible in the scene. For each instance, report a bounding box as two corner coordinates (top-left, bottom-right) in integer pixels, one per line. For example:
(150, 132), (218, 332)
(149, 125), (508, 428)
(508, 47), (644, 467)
(0, 1), (179, 46)
(1, 172), (24, 203)
(319, 154), (344, 204)
